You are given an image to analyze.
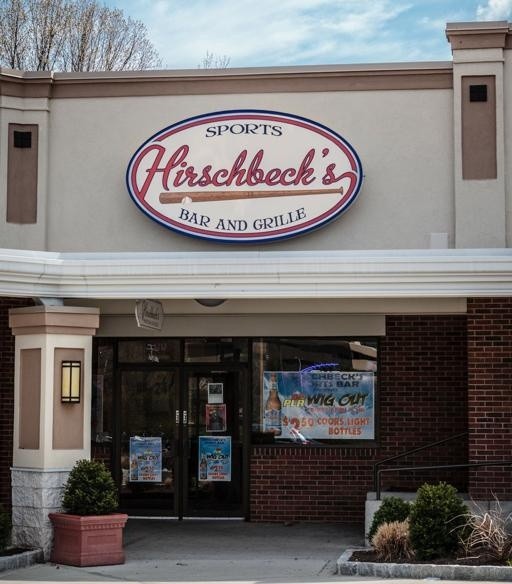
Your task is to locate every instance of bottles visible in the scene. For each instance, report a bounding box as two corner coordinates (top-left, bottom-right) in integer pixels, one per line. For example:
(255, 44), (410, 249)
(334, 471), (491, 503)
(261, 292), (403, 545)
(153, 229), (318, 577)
(265, 374), (281, 436)
(200, 453), (207, 479)
(131, 450), (138, 480)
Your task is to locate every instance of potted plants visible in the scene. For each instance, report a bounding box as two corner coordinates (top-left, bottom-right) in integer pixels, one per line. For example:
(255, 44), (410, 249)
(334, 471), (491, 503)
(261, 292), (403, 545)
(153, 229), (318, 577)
(48, 460), (128, 566)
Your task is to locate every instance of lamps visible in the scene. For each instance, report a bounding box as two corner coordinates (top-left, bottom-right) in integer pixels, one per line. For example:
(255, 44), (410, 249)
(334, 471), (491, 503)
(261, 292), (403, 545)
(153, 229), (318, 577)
(61, 360), (81, 402)
